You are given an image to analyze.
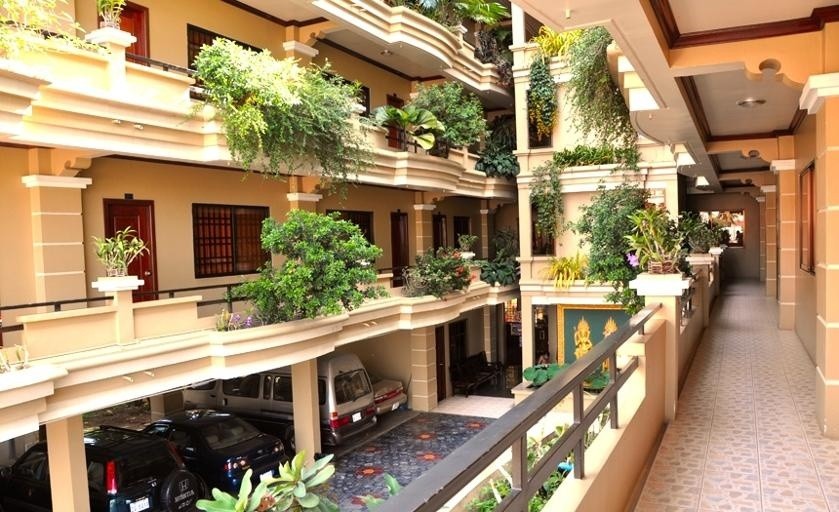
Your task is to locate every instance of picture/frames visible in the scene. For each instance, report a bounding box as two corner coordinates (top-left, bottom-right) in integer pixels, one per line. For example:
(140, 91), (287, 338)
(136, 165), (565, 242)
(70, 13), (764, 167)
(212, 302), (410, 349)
(556, 304), (635, 387)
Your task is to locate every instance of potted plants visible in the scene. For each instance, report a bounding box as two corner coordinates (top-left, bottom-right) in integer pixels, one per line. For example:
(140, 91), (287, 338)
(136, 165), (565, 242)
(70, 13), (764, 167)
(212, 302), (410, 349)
(90, 225), (148, 278)
(458, 234), (479, 251)
(626, 208), (722, 274)
(97, 0), (127, 29)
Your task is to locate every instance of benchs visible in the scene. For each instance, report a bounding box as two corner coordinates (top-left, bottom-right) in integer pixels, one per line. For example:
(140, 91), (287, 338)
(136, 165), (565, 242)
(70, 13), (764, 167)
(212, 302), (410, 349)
(453, 351), (502, 397)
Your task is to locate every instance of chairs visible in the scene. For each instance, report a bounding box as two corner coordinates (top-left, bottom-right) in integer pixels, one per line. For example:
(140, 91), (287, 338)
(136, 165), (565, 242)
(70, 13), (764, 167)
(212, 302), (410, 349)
(237, 376), (256, 396)
(205, 424), (245, 444)
